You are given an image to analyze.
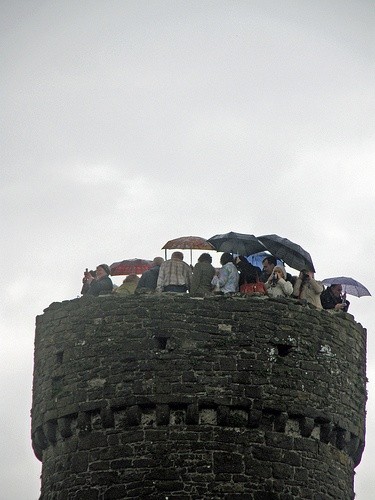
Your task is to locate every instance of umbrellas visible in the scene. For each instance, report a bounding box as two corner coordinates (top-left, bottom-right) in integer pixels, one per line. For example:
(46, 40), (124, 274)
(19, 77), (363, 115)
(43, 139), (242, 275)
(320, 277), (372, 298)
(109, 258), (153, 276)
(207, 231), (267, 258)
(258, 233), (315, 272)
(161, 236), (216, 265)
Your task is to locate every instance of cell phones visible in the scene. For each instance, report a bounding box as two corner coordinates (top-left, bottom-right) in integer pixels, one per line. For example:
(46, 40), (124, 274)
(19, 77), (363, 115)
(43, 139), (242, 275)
(86, 268), (88, 272)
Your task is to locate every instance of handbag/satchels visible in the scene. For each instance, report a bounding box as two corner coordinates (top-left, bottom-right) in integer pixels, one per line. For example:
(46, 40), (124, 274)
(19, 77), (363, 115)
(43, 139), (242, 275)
(241, 282), (268, 295)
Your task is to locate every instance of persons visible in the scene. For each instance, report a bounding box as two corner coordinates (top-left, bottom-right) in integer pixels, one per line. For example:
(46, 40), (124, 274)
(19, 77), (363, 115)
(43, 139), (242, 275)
(82, 252), (351, 312)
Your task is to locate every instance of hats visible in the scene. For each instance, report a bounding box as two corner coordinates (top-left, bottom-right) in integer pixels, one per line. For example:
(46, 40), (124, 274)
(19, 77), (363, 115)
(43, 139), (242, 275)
(99, 264), (110, 275)
(221, 253), (232, 263)
(274, 267), (282, 272)
